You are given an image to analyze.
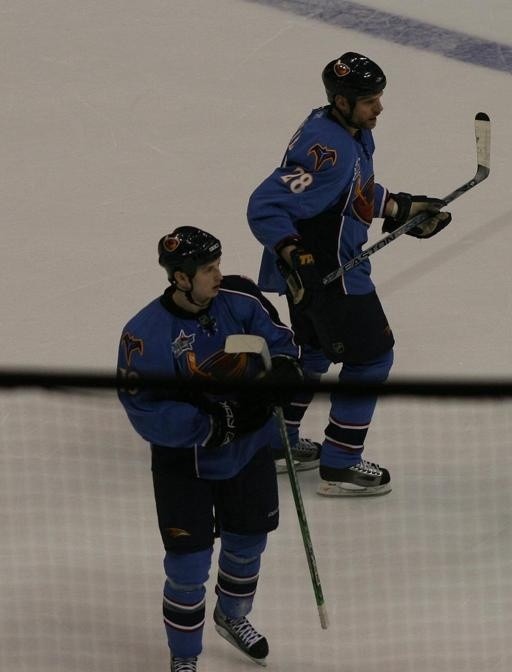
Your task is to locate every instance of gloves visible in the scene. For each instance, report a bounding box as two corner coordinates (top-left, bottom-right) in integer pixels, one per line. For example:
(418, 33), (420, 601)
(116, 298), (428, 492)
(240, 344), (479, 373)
(382, 192), (451, 239)
(202, 391), (272, 450)
(243, 353), (304, 405)
(275, 234), (324, 306)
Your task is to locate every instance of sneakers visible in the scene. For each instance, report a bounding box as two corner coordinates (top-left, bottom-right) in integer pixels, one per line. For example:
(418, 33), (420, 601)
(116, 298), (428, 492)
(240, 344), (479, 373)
(275, 438), (321, 462)
(170, 656), (197, 672)
(214, 602), (269, 658)
(319, 459), (390, 487)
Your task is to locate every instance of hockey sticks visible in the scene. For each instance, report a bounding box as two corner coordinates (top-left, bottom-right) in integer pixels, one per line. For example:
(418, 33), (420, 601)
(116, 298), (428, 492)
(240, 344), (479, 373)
(323, 112), (492, 289)
(224, 335), (329, 629)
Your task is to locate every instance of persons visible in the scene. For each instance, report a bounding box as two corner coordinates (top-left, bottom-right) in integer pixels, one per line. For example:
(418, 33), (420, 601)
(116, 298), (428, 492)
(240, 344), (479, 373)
(113, 224), (310, 671)
(246, 49), (453, 488)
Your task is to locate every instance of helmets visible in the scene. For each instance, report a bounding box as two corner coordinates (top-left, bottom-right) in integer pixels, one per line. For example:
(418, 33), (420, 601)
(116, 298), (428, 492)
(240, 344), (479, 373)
(158, 226), (222, 270)
(322, 51), (386, 102)
